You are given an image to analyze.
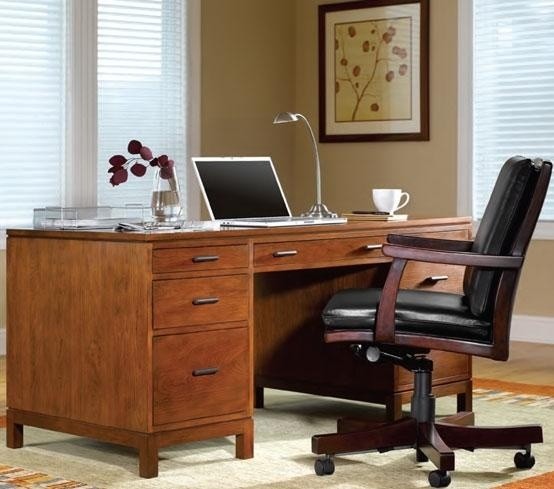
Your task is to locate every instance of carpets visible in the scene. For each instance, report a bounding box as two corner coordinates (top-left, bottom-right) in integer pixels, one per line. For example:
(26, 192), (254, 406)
(1, 375), (554, 487)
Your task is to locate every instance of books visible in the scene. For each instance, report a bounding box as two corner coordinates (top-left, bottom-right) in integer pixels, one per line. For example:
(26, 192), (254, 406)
(341, 213), (408, 222)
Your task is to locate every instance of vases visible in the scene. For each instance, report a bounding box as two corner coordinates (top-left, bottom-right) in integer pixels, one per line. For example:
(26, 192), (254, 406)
(144, 166), (188, 231)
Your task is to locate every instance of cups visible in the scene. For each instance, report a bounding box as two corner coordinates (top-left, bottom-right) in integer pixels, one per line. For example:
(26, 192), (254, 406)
(370, 187), (410, 215)
(141, 207), (159, 229)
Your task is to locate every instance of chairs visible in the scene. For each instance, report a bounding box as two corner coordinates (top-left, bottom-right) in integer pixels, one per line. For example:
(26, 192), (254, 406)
(311, 154), (554, 485)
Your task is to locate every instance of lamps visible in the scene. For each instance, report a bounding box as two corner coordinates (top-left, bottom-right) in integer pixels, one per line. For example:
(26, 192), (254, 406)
(271, 110), (350, 226)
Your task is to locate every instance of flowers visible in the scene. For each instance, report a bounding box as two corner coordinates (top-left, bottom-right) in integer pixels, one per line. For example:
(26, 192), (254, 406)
(105, 138), (176, 188)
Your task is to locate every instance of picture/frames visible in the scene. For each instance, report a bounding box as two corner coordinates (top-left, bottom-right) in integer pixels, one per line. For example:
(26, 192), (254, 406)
(318, 0), (429, 143)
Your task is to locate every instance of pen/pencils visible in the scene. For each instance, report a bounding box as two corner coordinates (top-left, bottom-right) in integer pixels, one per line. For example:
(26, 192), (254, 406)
(352, 210), (390, 215)
(144, 227), (180, 229)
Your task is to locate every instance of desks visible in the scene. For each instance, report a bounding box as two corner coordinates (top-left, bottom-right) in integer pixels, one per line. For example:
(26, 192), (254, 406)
(4, 215), (475, 478)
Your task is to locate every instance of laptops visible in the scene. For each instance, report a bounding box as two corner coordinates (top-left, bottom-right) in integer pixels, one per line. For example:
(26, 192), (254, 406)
(191, 154), (348, 228)
